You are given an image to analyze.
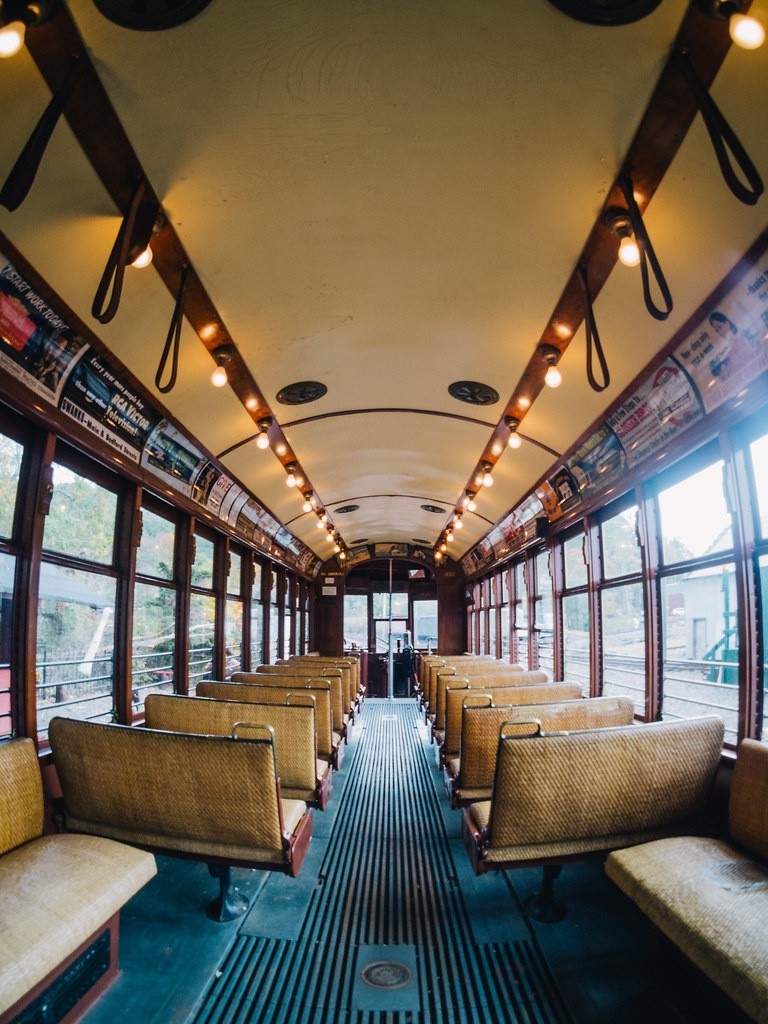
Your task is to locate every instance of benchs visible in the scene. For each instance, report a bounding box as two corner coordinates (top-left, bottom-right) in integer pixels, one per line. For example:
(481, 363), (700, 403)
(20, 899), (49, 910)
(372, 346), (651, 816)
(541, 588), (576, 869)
(410, 650), (768, 1024)
(0, 654), (366, 1024)
